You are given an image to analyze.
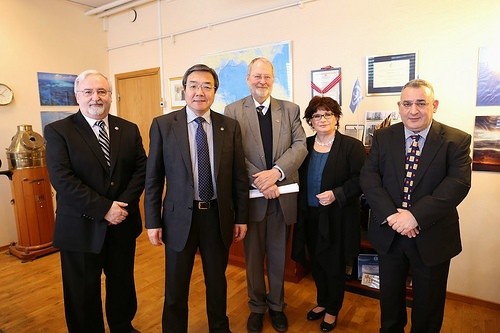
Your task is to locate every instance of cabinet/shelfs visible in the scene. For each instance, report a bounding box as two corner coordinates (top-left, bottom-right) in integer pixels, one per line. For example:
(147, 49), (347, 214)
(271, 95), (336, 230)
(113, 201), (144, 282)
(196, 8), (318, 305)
(345, 240), (414, 305)
(196, 223), (307, 284)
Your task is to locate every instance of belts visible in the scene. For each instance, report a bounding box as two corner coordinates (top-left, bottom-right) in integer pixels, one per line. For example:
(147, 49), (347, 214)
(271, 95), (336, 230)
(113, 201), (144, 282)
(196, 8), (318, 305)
(194, 199), (218, 210)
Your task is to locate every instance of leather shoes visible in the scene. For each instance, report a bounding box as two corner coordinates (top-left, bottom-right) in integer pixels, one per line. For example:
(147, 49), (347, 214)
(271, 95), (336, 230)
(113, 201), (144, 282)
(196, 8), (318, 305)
(320, 316), (337, 333)
(247, 312), (265, 333)
(307, 304), (326, 320)
(268, 308), (288, 332)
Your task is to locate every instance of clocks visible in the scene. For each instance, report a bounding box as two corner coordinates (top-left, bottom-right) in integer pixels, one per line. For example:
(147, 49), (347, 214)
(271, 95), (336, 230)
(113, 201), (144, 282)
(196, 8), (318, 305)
(0, 83), (14, 106)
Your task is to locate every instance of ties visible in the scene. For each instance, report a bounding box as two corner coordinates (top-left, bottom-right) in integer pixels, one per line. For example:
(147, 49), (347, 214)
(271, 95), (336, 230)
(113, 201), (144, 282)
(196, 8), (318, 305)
(193, 117), (214, 205)
(94, 120), (110, 167)
(256, 106), (265, 115)
(402, 136), (421, 210)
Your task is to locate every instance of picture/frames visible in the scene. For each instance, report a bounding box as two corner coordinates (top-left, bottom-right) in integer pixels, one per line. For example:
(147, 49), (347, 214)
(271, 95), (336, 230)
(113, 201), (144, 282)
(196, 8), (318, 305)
(366, 51), (418, 96)
(345, 125), (366, 142)
(168, 77), (187, 110)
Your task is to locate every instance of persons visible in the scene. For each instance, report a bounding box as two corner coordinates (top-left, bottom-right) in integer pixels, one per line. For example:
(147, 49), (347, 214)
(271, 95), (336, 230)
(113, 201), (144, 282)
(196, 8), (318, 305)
(46, 71), (149, 333)
(290, 96), (367, 332)
(224, 57), (308, 333)
(360, 79), (472, 333)
(144, 64), (246, 333)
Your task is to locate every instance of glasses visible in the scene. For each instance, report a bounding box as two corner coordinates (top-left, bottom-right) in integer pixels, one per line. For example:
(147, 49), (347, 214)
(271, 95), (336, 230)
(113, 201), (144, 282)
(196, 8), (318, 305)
(184, 82), (216, 91)
(400, 100), (433, 109)
(310, 113), (334, 120)
(77, 89), (112, 96)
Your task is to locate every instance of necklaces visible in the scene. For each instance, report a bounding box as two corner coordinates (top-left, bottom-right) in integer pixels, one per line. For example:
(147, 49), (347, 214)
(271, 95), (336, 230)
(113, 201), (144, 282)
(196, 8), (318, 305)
(315, 135), (335, 146)
